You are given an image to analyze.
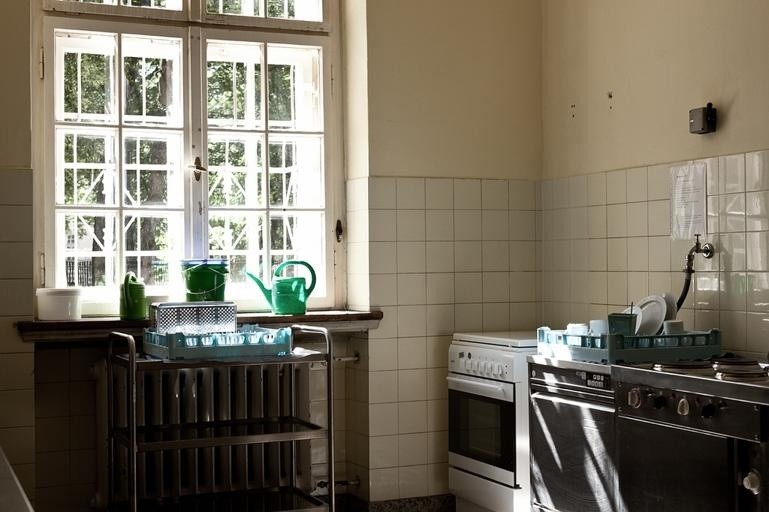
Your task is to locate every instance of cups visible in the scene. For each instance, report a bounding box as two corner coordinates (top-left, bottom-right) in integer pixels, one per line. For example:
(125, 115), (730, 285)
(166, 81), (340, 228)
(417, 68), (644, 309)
(565, 319), (607, 335)
(664, 320), (683, 334)
(608, 313), (637, 336)
(623, 333), (716, 349)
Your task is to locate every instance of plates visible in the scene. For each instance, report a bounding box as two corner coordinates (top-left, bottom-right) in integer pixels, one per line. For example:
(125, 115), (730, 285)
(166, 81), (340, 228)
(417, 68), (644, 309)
(622, 295), (667, 335)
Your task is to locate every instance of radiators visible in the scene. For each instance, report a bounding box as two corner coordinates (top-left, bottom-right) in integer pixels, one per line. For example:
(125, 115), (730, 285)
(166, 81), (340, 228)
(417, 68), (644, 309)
(88, 352), (311, 511)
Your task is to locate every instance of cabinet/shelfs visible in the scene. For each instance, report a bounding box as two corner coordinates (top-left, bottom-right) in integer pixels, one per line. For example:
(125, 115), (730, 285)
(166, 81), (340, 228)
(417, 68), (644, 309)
(107, 324), (336, 512)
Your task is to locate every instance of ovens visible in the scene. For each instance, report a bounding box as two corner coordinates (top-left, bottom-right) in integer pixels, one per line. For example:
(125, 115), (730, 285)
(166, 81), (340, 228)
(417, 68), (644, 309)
(444, 370), (526, 511)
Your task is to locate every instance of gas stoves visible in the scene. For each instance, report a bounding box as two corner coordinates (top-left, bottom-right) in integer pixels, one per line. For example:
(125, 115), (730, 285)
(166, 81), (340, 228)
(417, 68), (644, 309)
(613, 351), (769, 443)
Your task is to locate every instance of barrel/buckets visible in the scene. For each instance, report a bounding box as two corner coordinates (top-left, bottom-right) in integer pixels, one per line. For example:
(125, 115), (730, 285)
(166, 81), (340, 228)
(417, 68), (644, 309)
(179, 258), (232, 302)
